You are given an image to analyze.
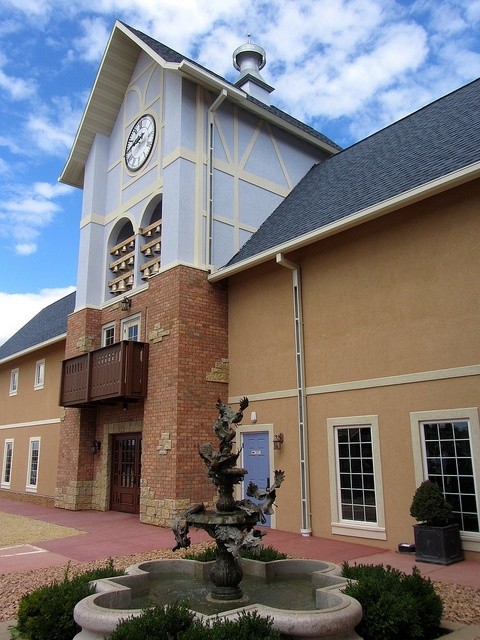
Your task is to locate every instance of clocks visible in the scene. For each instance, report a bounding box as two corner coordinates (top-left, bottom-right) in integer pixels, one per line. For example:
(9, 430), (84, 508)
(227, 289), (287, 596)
(123, 114), (156, 172)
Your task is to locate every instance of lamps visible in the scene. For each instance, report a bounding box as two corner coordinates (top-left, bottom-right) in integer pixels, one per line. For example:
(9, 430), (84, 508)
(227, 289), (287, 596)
(88, 439), (101, 454)
(120, 296), (131, 311)
(273, 433), (283, 449)
